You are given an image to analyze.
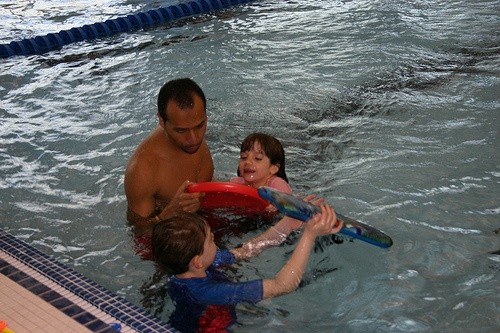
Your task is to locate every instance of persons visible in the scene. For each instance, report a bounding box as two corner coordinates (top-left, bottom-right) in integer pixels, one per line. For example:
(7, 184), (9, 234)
(124, 79), (214, 248)
(224, 133), (296, 223)
(151, 194), (344, 333)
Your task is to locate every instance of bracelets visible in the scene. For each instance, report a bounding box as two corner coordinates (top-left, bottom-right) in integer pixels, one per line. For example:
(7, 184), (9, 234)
(154, 215), (162, 223)
